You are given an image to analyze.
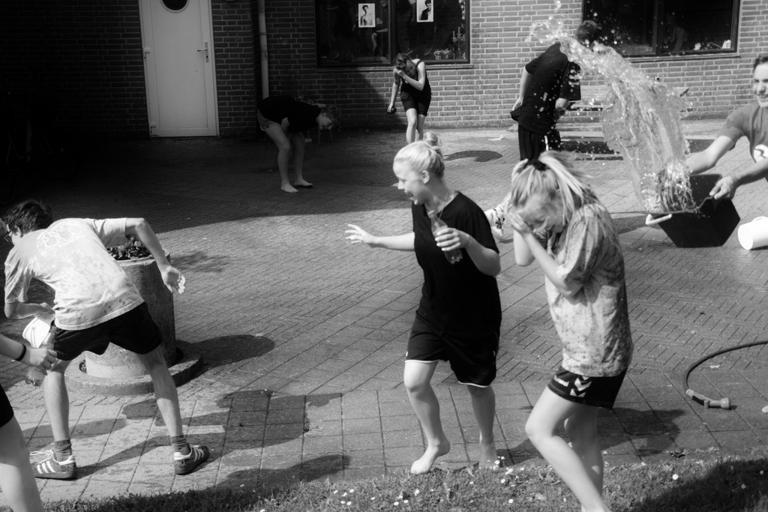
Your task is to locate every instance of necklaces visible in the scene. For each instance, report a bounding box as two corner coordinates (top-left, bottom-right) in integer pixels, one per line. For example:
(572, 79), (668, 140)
(425, 191), (451, 219)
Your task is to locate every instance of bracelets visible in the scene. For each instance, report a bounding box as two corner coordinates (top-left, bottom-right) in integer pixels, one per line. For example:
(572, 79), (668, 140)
(14, 343), (27, 362)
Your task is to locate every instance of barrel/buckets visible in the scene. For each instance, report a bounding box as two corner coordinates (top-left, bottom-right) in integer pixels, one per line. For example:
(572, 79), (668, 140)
(646, 175), (741, 248)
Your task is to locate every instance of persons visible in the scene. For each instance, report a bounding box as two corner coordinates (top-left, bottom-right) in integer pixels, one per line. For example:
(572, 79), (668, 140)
(0, 334), (58, 512)
(509, 19), (602, 167)
(505, 151), (634, 511)
(0, 198), (208, 484)
(387, 51), (432, 143)
(341, 131), (506, 476)
(654, 53), (768, 202)
(418, 0), (432, 20)
(257, 93), (341, 194)
(360, 6), (372, 26)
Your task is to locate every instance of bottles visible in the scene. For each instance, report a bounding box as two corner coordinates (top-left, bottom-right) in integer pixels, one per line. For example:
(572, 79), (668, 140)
(424, 206), (464, 265)
(24, 324), (61, 388)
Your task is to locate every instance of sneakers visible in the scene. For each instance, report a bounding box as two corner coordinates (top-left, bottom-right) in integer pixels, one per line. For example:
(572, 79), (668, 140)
(30, 450), (77, 479)
(174, 446), (210, 475)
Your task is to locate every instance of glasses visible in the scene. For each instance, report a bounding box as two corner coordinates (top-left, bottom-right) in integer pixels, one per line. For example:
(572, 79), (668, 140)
(2, 230), (13, 243)
